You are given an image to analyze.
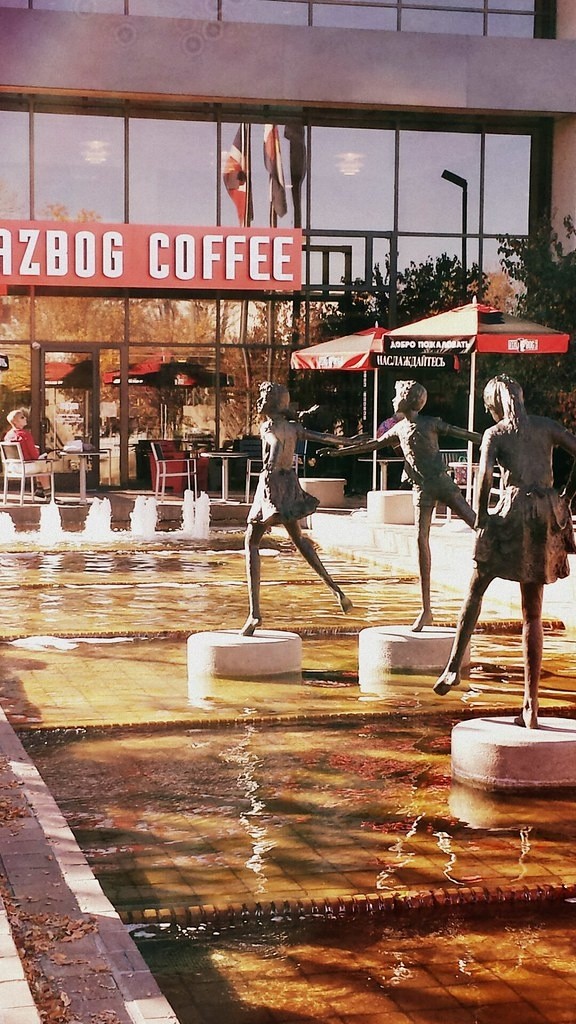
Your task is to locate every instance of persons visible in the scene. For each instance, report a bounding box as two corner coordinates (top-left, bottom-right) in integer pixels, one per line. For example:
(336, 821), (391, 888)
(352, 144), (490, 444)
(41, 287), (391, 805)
(378, 411), (411, 489)
(433, 375), (576, 728)
(239, 381), (369, 636)
(317, 380), (482, 632)
(3, 410), (64, 504)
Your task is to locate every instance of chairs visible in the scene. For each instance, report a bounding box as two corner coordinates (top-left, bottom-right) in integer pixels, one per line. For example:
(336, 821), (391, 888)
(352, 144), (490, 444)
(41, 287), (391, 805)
(430, 449), (467, 524)
(0, 441), (55, 506)
(245, 457), (262, 504)
(150, 442), (197, 502)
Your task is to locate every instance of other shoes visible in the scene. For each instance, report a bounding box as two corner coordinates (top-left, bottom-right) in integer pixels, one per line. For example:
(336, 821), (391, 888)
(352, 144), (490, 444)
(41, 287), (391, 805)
(35, 487), (47, 500)
(45, 493), (61, 503)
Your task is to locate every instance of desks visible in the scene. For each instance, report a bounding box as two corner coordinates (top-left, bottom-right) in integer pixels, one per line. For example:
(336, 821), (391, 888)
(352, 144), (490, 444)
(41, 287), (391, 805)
(358, 456), (405, 490)
(448, 462), (499, 511)
(57, 450), (108, 506)
(201, 452), (249, 505)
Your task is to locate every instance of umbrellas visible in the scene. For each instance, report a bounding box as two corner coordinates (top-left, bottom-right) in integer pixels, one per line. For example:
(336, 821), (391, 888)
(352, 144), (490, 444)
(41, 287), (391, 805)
(0, 353), (9, 370)
(27, 363), (90, 458)
(104, 351), (235, 440)
(382, 295), (569, 506)
(291, 322), (460, 492)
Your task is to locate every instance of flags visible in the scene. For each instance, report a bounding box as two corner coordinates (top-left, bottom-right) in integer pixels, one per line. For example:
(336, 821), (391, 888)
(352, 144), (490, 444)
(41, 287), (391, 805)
(285, 124), (307, 203)
(263, 124), (287, 217)
(223, 125), (254, 228)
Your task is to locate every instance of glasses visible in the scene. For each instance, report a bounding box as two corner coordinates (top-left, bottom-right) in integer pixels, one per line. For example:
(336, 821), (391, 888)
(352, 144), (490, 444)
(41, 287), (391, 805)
(15, 415), (24, 420)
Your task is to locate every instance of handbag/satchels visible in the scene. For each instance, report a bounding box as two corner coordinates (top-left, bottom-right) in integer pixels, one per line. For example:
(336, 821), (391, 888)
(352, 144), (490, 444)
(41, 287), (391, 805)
(453, 456), (479, 485)
